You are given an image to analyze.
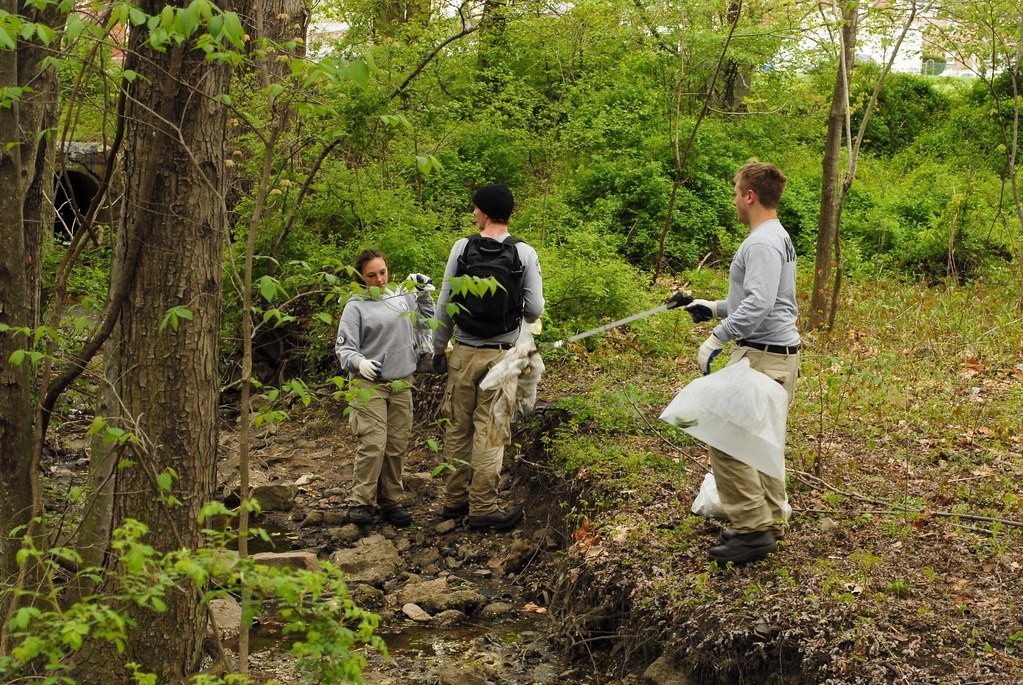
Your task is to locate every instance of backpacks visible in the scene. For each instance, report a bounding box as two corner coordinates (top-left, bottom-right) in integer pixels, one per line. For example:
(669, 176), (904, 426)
(448, 233), (531, 339)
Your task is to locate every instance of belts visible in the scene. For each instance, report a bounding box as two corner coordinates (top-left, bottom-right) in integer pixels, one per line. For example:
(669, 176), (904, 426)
(735, 338), (798, 354)
(455, 340), (513, 350)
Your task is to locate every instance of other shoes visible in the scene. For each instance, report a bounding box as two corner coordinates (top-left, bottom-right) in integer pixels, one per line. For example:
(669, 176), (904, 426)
(721, 529), (785, 545)
(469, 505), (526, 531)
(349, 505), (374, 524)
(708, 529), (777, 566)
(380, 507), (411, 526)
(441, 504), (468, 520)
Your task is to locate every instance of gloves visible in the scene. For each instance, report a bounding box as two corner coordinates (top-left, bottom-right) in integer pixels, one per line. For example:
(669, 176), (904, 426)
(412, 273), (433, 290)
(698, 332), (725, 375)
(432, 354), (449, 374)
(683, 299), (718, 323)
(358, 358), (383, 382)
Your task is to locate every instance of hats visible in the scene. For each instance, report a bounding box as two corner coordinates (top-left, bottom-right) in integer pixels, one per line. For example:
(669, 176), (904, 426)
(473, 184), (515, 221)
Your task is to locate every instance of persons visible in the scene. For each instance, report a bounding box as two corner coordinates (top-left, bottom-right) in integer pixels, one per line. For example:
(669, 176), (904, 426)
(333, 249), (434, 525)
(685, 161), (803, 567)
(432, 187), (546, 531)
